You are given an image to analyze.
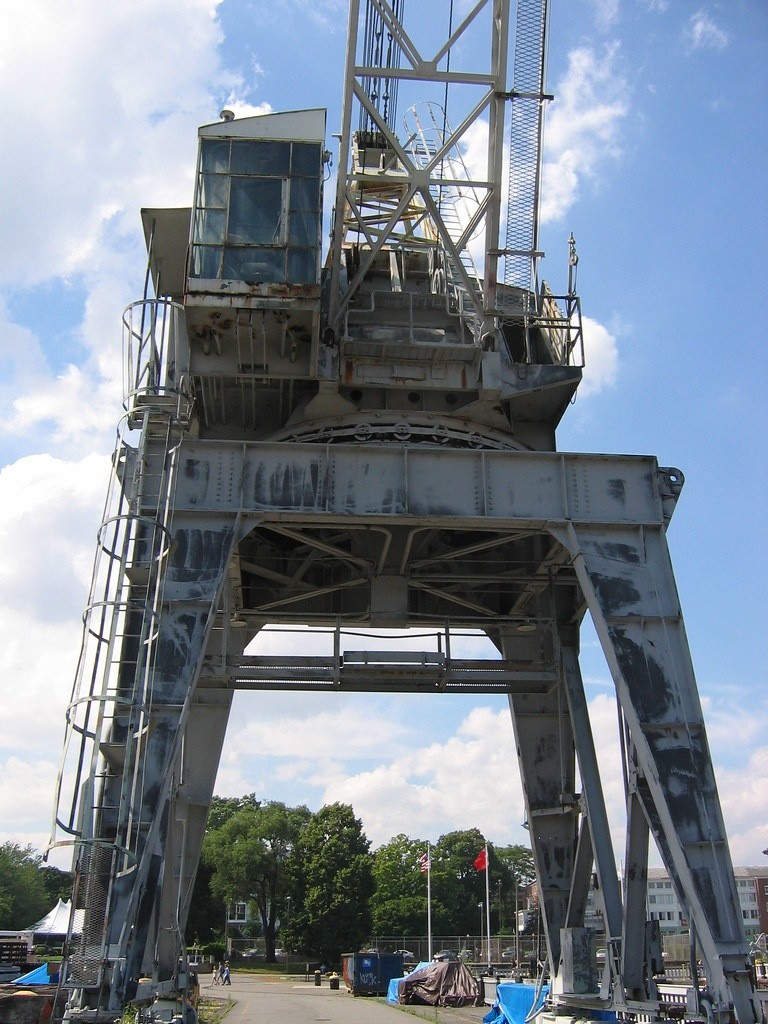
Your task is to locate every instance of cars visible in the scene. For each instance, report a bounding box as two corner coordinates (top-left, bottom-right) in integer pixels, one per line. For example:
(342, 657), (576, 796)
(596, 948), (607, 960)
(457, 949), (473, 960)
(475, 945), (543, 964)
(393, 950), (415, 961)
(275, 948), (288, 959)
(434, 950), (455, 960)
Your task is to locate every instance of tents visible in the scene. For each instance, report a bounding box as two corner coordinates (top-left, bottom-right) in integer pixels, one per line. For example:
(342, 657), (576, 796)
(23, 898), (85, 945)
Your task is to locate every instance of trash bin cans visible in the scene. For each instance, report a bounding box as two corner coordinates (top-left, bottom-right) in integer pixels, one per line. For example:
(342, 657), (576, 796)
(342, 951), (405, 998)
(314, 970), (322, 986)
(328, 972), (339, 990)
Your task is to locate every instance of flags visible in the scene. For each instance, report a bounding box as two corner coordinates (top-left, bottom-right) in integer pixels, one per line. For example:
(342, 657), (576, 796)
(474, 849), (488, 871)
(419, 852), (430, 871)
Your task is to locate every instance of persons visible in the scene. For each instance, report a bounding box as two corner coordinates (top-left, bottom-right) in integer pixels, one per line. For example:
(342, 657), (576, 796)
(210, 961), (231, 986)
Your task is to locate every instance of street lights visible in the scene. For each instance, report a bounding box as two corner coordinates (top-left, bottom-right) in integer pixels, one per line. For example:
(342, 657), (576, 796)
(478, 902), (485, 954)
(285, 896), (292, 974)
(514, 871), (521, 973)
(497, 879), (503, 962)
